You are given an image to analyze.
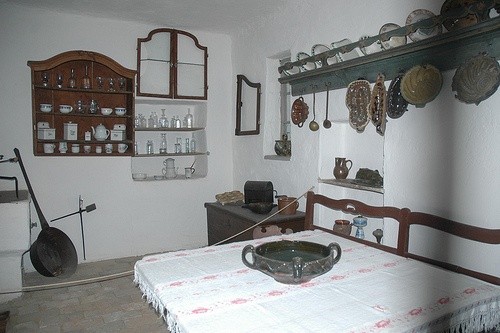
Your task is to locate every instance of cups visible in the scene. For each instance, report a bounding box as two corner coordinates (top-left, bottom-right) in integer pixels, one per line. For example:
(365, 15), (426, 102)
(163, 158), (195, 178)
(277, 197), (299, 215)
(37, 75), (128, 154)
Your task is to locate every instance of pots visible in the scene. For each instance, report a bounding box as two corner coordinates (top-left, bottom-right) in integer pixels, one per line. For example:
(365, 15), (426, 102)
(292, 97), (309, 127)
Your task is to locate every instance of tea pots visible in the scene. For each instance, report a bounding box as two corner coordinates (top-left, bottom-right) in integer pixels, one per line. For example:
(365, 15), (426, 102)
(275, 134), (291, 156)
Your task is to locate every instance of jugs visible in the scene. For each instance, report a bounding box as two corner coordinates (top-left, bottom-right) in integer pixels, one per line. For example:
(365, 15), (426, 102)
(162, 167), (179, 178)
(333, 157), (352, 181)
(90, 122), (110, 142)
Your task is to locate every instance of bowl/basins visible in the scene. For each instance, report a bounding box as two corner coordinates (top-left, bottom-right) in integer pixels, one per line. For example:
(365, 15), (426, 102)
(242, 240), (341, 284)
(132, 173), (164, 180)
(246, 202), (277, 215)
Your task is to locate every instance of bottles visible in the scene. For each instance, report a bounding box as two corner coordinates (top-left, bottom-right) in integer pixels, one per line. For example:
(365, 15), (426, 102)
(39, 64), (95, 88)
(353, 216), (367, 240)
(135, 107), (197, 155)
(334, 220), (352, 236)
(372, 228), (383, 245)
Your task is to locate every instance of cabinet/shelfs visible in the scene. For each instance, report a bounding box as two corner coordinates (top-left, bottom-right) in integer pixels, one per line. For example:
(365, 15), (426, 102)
(135, 127), (205, 157)
(204, 202), (305, 246)
(27, 50), (137, 157)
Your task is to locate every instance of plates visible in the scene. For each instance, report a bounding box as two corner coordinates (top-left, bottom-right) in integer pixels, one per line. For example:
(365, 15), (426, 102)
(386, 56), (500, 119)
(406, 0), (500, 42)
(331, 39), (361, 61)
(281, 44), (338, 76)
(359, 34), (383, 55)
(379, 22), (407, 50)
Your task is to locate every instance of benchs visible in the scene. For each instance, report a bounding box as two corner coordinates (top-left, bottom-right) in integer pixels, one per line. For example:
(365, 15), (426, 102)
(305, 191), (500, 287)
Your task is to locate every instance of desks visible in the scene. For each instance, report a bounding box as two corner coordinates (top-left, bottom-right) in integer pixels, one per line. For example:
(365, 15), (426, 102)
(134, 229), (500, 333)
